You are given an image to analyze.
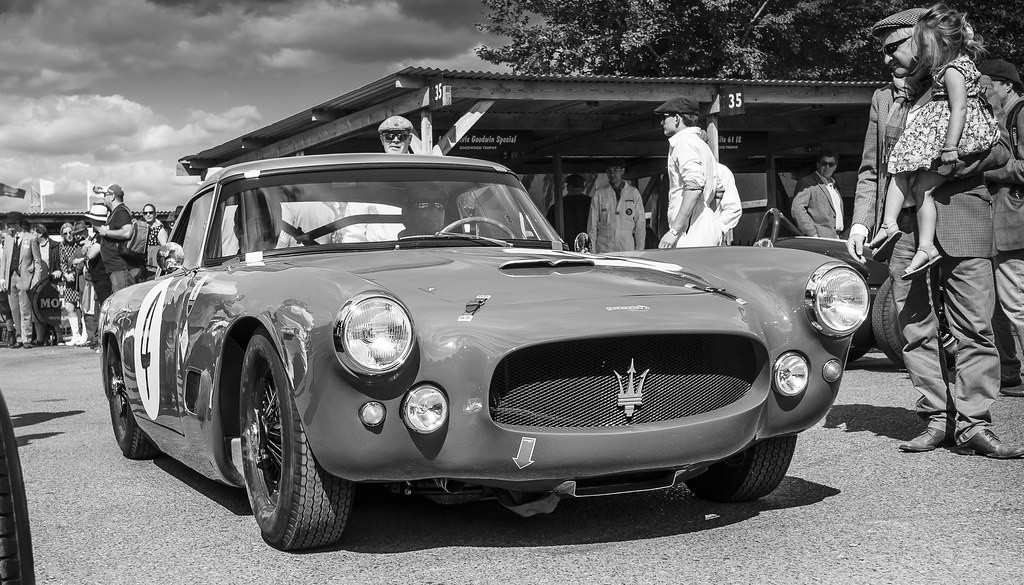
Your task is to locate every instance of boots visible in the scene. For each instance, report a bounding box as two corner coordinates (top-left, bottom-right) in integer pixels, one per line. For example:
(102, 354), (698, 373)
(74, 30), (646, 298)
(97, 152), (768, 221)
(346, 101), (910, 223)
(75, 316), (87, 345)
(65, 317), (81, 345)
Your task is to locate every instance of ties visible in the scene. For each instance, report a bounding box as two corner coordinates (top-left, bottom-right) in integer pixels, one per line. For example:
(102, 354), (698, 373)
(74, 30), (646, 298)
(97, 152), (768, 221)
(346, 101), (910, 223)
(16, 236), (20, 246)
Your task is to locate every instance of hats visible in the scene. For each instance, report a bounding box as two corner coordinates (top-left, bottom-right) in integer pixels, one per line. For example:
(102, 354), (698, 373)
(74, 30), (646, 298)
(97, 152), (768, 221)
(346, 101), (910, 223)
(5, 211), (25, 223)
(85, 205), (108, 220)
(975, 60), (1022, 83)
(565, 174), (585, 183)
(378, 115), (413, 133)
(71, 221), (90, 234)
(98, 184), (123, 195)
(872, 8), (930, 39)
(654, 97), (699, 115)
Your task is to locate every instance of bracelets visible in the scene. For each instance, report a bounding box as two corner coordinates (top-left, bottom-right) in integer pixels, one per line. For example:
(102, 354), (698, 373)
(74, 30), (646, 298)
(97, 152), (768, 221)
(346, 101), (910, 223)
(937, 146), (961, 153)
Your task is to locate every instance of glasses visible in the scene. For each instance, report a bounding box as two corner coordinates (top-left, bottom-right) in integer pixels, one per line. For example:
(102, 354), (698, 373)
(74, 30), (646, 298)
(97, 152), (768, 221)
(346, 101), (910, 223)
(818, 161), (836, 167)
(663, 113), (676, 120)
(383, 134), (411, 140)
(418, 200), (445, 210)
(883, 35), (914, 56)
(143, 212), (154, 214)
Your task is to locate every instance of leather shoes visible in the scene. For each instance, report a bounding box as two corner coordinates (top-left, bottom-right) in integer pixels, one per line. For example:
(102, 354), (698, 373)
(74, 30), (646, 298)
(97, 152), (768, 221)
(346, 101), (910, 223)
(899, 427), (957, 451)
(958, 428), (1024, 459)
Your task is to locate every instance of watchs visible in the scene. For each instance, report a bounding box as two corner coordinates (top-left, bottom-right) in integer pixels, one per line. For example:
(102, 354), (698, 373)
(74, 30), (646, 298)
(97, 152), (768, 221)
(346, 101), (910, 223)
(104, 231), (109, 236)
(670, 228), (678, 235)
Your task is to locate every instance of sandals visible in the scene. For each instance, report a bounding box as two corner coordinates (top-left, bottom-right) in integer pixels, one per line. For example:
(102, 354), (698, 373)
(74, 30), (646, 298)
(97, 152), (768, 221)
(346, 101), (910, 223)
(901, 245), (942, 280)
(872, 223), (902, 260)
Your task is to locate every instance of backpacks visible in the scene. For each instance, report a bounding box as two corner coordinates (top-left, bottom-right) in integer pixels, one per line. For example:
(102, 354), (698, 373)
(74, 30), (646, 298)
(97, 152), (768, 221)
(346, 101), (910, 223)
(107, 206), (150, 258)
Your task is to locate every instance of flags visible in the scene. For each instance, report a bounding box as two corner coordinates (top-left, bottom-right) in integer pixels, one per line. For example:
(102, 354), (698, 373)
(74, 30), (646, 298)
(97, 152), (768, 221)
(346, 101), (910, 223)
(89, 182), (108, 199)
(40, 179), (55, 196)
(31, 186), (45, 208)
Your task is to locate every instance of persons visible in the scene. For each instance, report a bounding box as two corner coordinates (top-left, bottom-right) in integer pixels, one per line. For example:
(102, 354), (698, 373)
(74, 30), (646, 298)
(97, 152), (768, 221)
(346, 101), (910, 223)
(586, 158), (646, 253)
(790, 150), (845, 240)
(0, 205), (110, 351)
(398, 182), (450, 240)
(547, 174), (592, 251)
(99, 184), (184, 293)
(234, 195), (282, 255)
(653, 95), (742, 251)
(846, 0), (1024, 459)
(378, 116), (414, 154)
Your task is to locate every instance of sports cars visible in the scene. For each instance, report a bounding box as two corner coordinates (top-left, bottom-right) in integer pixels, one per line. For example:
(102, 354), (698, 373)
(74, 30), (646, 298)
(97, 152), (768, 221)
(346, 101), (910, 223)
(99, 152), (899, 554)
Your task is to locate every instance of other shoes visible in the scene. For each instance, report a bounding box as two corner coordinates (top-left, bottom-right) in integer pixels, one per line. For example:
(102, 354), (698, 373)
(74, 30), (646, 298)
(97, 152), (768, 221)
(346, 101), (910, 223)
(1001, 383), (1024, 397)
(82, 341), (97, 346)
(6, 333), (65, 349)
